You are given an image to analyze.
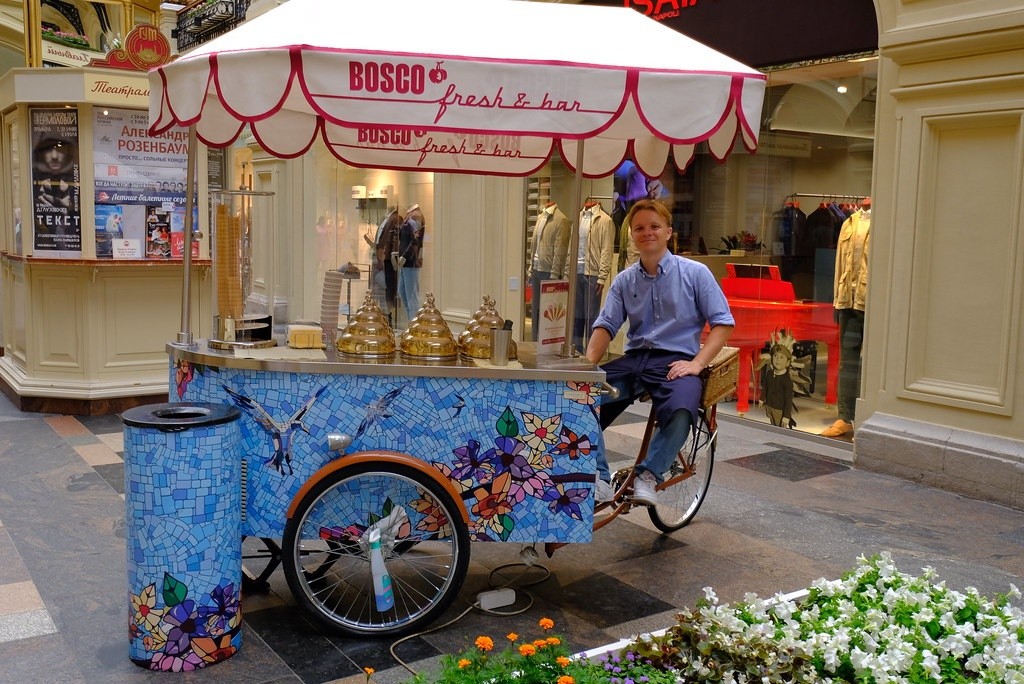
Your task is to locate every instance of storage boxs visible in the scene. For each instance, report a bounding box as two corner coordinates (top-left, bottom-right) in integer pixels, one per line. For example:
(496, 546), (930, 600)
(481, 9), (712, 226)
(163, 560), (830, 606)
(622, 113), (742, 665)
(699, 343), (740, 407)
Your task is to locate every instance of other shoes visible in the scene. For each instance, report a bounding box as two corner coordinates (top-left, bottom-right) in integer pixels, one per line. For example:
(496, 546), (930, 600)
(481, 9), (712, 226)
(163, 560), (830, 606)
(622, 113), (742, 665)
(821, 419), (854, 437)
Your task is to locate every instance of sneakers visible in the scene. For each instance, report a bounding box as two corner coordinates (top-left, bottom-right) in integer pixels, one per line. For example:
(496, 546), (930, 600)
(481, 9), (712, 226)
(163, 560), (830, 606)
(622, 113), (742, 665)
(594, 471), (615, 502)
(633, 474), (658, 507)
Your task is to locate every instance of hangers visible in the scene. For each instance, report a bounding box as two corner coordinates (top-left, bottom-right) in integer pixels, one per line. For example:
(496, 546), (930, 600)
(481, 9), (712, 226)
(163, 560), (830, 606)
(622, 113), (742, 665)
(786, 194), (801, 211)
(818, 192), (871, 212)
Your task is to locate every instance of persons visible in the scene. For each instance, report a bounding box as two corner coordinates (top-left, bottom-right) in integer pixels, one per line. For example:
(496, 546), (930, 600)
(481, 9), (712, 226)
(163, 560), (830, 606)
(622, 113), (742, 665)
(147, 207), (159, 223)
(821, 199), (872, 442)
(32, 124), (80, 248)
(194, 183), (197, 193)
(525, 202), (571, 342)
(586, 200), (735, 505)
(372, 204), (425, 329)
(564, 202), (615, 355)
(316, 216), (346, 269)
(759, 344), (793, 428)
(143, 181), (187, 193)
(149, 226), (169, 252)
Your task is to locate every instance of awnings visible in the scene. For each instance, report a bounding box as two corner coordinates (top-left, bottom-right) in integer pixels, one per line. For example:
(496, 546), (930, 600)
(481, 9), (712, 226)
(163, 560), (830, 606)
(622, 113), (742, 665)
(147, 1), (767, 359)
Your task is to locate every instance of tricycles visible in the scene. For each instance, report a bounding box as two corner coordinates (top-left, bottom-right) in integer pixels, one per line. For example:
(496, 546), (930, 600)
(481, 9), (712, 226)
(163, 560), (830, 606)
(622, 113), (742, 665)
(147, 0), (717, 636)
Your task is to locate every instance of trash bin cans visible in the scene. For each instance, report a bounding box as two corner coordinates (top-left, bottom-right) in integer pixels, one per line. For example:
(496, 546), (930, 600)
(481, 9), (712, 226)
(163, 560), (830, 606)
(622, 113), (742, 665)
(121, 400), (246, 671)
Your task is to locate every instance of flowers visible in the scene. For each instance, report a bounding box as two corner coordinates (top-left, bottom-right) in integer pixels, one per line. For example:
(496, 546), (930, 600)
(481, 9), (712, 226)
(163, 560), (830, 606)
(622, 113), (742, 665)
(42, 26), (90, 46)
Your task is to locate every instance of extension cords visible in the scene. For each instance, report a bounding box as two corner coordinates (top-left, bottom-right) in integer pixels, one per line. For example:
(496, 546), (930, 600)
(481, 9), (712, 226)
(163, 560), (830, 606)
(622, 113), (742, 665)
(476, 588), (516, 609)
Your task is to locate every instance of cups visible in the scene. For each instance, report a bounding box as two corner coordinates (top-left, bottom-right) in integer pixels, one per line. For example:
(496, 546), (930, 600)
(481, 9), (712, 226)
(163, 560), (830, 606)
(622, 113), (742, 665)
(320, 271), (344, 344)
(490, 327), (513, 367)
